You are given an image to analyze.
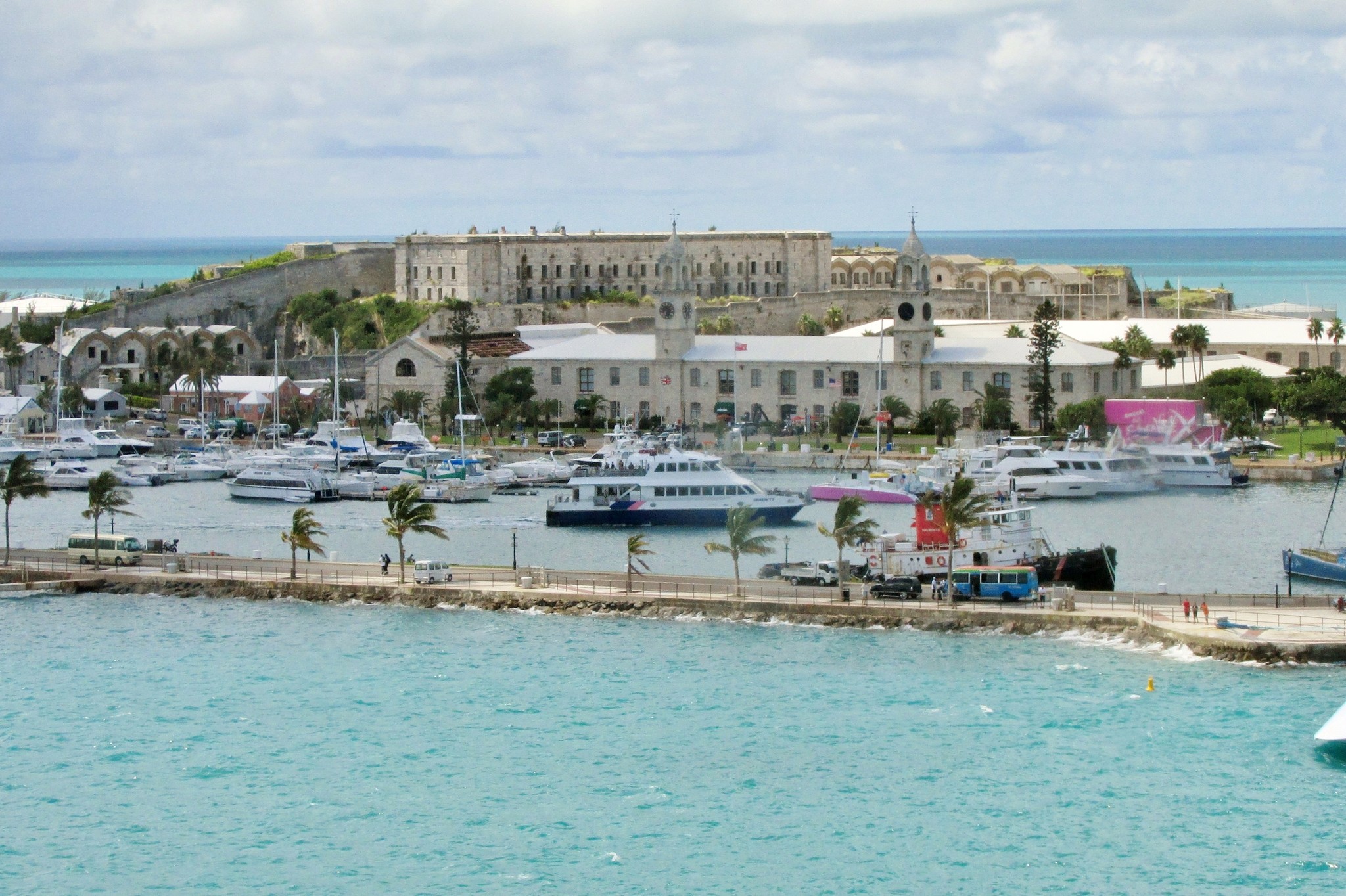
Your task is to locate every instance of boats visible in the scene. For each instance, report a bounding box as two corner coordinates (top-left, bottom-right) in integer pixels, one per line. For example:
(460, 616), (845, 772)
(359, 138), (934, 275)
(853, 462), (1117, 594)
(915, 407), (1288, 502)
(546, 407), (817, 529)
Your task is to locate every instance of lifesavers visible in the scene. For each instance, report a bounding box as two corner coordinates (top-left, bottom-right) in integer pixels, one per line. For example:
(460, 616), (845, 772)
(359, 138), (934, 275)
(959, 538), (967, 548)
(869, 555), (877, 567)
(937, 556), (946, 566)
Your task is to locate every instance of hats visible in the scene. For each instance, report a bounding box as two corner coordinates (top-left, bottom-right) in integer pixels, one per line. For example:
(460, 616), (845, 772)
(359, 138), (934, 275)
(933, 577), (936, 579)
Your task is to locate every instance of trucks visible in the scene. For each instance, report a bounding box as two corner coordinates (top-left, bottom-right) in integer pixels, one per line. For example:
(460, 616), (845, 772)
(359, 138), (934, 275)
(780, 559), (840, 586)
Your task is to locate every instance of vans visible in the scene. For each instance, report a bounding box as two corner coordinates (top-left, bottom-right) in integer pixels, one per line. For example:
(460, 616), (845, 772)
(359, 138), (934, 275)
(143, 408), (167, 422)
(177, 417), (257, 440)
(413, 559), (453, 584)
(537, 430), (565, 448)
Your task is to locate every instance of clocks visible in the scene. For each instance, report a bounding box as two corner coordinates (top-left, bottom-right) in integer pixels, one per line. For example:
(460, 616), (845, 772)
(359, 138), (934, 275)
(923, 303), (932, 321)
(683, 301), (692, 319)
(659, 302), (675, 320)
(898, 302), (914, 320)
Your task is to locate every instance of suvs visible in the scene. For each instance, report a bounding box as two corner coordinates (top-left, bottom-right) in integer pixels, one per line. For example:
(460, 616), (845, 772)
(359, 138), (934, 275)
(869, 575), (923, 600)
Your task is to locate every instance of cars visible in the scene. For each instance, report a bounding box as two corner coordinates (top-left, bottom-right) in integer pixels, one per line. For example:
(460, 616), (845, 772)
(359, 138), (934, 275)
(294, 427), (316, 439)
(145, 426), (171, 438)
(260, 423), (292, 440)
(603, 429), (684, 449)
(732, 420), (756, 438)
(562, 433), (587, 447)
(121, 420), (144, 430)
(1262, 408), (1278, 427)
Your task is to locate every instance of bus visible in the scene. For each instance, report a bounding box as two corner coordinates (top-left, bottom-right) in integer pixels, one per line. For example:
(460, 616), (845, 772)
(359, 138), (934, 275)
(67, 532), (143, 566)
(941, 565), (1040, 603)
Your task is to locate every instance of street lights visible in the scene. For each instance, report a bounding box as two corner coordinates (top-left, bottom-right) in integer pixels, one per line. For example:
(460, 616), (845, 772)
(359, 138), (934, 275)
(510, 526), (518, 570)
(804, 407), (808, 436)
(783, 534), (790, 581)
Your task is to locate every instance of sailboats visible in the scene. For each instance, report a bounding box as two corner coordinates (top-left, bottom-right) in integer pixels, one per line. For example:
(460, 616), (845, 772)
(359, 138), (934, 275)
(1281, 444), (1346, 587)
(0, 304), (726, 506)
(808, 313), (921, 505)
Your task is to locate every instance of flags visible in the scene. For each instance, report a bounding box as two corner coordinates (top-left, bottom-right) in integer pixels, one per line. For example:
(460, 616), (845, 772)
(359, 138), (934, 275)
(735, 342), (747, 351)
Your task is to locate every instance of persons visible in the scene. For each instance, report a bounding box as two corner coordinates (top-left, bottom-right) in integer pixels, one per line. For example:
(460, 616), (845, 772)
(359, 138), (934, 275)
(379, 553), (391, 576)
(603, 489), (608, 504)
(860, 581), (868, 605)
(1191, 602), (1199, 623)
(972, 574), (979, 596)
(997, 490), (1003, 504)
(1182, 598), (1190, 622)
(931, 577), (945, 600)
(407, 554), (414, 562)
(1030, 584), (1046, 609)
(1200, 602), (1209, 625)
(597, 460), (634, 477)
(403, 550), (405, 561)
(950, 584), (959, 609)
